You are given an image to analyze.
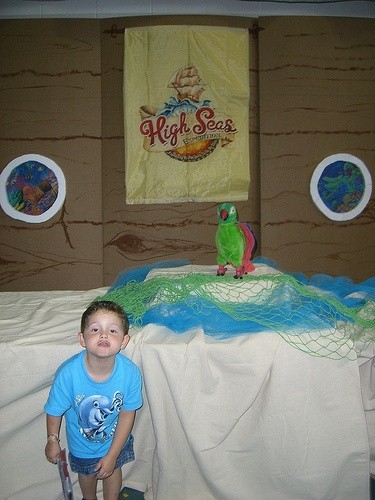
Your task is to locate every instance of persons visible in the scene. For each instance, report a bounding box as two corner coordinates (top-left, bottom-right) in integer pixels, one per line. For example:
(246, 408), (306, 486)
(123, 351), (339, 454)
(45, 300), (145, 498)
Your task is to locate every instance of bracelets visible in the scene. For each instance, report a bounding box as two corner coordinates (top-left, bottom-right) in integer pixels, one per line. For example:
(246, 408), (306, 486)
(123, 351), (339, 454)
(47, 433), (62, 443)
